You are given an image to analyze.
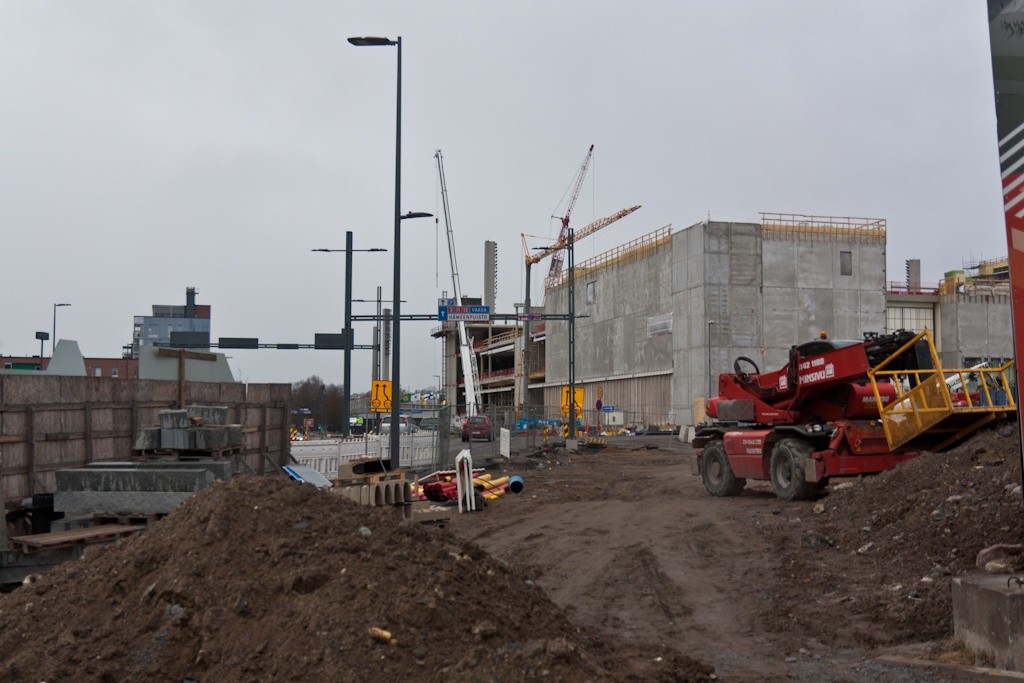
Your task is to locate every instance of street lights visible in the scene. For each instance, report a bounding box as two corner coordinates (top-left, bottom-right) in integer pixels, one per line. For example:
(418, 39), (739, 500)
(52, 303), (72, 356)
(432, 375), (441, 398)
(311, 231), (388, 441)
(35, 331), (50, 370)
(346, 34), (435, 478)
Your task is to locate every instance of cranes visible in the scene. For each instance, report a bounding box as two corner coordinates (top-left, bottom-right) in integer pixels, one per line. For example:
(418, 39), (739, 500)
(520, 144), (642, 296)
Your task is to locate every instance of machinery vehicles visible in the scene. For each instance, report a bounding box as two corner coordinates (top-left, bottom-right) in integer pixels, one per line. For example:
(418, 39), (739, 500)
(673, 328), (1015, 504)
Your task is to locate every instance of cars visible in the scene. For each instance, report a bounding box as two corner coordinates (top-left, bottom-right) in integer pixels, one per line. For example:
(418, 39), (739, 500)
(463, 416), (497, 441)
(380, 413), (419, 434)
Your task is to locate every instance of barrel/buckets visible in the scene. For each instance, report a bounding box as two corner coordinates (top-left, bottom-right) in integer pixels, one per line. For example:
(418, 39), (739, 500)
(509, 476), (525, 494)
